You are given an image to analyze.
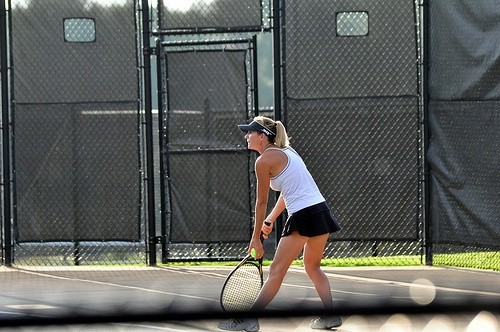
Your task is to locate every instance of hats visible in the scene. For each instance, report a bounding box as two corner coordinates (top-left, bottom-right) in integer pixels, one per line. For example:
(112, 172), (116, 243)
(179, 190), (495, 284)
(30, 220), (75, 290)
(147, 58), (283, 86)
(238, 120), (276, 139)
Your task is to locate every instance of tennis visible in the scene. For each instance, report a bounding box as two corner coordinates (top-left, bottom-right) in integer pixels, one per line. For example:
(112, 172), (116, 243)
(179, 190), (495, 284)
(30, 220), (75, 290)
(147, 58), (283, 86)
(250, 248), (262, 258)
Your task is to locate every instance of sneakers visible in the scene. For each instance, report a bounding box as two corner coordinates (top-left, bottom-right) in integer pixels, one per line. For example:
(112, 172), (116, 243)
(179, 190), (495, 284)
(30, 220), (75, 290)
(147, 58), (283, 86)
(309, 317), (342, 329)
(216, 318), (259, 332)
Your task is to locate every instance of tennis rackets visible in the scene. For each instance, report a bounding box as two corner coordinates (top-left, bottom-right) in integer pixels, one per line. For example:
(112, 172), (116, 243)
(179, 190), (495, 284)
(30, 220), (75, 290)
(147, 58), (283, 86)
(220, 217), (270, 312)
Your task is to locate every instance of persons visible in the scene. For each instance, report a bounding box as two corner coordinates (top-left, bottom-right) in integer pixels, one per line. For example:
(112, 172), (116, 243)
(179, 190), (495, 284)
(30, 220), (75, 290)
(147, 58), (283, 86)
(217, 116), (342, 332)
